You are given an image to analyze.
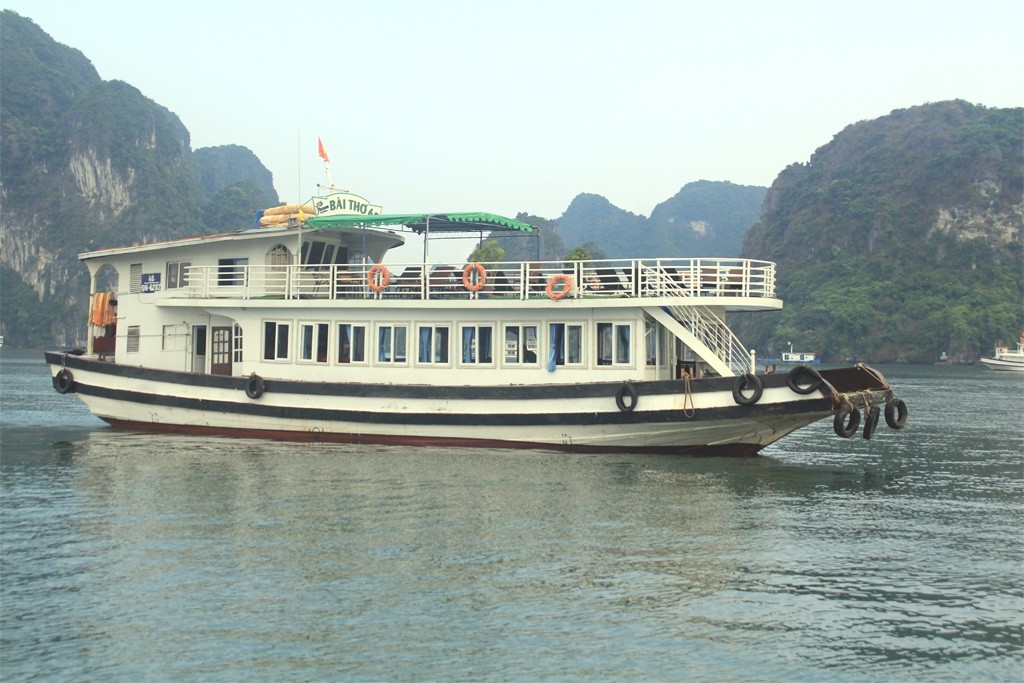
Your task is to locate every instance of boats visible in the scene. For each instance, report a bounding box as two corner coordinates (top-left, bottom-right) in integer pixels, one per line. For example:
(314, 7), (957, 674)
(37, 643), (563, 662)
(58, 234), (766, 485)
(45, 128), (893, 459)
(979, 331), (1024, 371)
(756, 341), (822, 366)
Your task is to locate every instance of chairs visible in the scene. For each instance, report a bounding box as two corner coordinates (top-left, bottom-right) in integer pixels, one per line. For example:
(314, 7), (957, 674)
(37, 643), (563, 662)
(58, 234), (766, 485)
(339, 266), (744, 294)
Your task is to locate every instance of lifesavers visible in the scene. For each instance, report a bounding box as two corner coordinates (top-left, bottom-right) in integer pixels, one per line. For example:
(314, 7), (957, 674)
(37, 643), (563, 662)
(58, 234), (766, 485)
(732, 373), (764, 406)
(245, 374), (266, 399)
(863, 405), (880, 439)
(462, 263), (487, 291)
(834, 403), (860, 438)
(786, 365), (823, 395)
(367, 265), (390, 291)
(885, 397), (907, 429)
(616, 381), (639, 413)
(54, 368), (73, 394)
(546, 274), (574, 300)
